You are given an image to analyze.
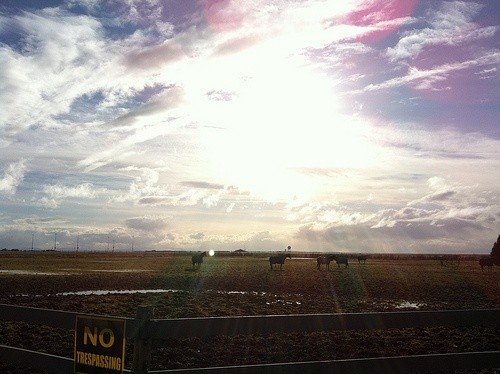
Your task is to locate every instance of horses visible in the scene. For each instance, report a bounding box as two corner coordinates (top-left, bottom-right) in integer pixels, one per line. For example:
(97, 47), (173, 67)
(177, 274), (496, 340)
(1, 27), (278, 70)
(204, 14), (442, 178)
(317, 255), (334, 271)
(479, 256), (498, 272)
(192, 251), (207, 267)
(333, 254), (349, 268)
(358, 255), (366, 264)
(269, 253), (291, 270)
